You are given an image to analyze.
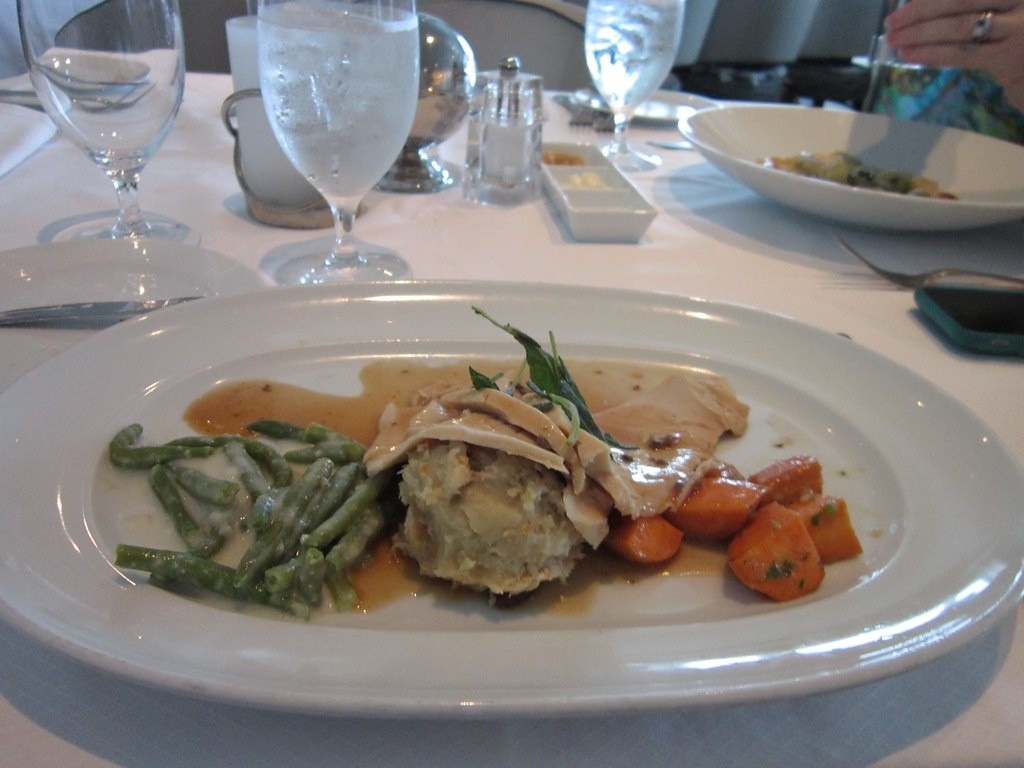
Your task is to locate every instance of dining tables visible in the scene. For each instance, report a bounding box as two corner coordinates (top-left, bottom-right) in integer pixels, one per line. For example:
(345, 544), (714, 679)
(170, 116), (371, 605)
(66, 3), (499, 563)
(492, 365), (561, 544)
(0, 70), (1024, 768)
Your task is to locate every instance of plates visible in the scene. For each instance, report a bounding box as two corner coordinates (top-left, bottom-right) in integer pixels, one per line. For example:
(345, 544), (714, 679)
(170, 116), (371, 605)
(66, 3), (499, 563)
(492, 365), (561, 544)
(676, 103), (1024, 230)
(553, 91), (721, 124)
(0, 295), (1023, 726)
(537, 142), (660, 243)
(0, 240), (266, 395)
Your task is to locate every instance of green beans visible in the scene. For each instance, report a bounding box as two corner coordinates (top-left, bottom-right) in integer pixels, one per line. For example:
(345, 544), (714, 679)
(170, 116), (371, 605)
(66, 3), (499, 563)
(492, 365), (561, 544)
(108, 420), (395, 618)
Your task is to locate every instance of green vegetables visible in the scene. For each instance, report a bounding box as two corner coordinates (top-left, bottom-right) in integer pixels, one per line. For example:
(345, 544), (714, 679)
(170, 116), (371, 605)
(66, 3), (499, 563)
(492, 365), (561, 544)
(463, 304), (639, 449)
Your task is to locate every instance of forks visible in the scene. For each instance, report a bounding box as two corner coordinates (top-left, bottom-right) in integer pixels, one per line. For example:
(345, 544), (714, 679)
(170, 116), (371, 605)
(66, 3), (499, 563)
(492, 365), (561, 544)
(833, 232), (1024, 298)
(1, 84), (170, 112)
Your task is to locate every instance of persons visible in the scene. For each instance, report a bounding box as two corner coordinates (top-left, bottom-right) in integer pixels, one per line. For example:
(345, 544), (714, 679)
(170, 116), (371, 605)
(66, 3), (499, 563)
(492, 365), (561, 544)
(872, 0), (1024, 147)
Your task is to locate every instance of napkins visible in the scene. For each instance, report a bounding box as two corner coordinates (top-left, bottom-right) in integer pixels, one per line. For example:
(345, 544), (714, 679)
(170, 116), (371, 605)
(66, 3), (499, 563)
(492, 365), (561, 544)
(0, 76), (71, 177)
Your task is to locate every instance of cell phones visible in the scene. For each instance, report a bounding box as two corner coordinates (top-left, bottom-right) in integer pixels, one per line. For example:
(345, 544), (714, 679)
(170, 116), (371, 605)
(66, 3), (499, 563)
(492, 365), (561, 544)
(913, 281), (1024, 360)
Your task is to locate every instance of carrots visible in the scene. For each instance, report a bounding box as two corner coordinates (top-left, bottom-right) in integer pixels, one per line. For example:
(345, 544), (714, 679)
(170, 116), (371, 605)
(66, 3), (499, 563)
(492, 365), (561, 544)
(601, 452), (863, 601)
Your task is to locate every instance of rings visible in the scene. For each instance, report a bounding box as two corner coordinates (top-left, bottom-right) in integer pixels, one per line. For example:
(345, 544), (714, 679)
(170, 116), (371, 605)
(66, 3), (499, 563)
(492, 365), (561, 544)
(972, 11), (993, 41)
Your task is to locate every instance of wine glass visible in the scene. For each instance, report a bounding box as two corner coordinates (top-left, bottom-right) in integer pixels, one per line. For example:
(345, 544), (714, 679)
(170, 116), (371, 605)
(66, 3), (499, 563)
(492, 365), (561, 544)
(17, 0), (201, 247)
(584, 0), (684, 174)
(255, 0), (416, 292)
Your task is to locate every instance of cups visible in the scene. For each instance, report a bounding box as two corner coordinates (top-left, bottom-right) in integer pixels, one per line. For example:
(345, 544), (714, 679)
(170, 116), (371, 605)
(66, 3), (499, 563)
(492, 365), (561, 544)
(862, 0), (996, 131)
(225, 16), (363, 229)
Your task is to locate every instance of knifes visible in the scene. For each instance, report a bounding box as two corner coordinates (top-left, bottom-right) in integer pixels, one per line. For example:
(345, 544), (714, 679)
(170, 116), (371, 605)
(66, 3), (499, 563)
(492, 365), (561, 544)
(646, 141), (693, 149)
(0, 297), (222, 327)
